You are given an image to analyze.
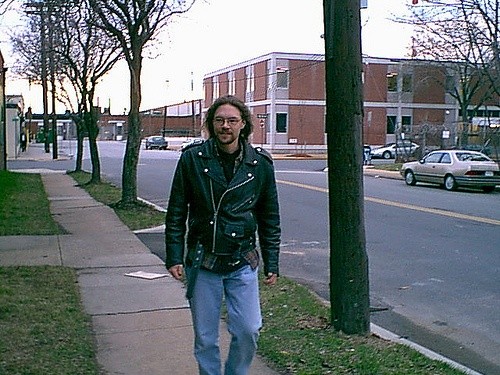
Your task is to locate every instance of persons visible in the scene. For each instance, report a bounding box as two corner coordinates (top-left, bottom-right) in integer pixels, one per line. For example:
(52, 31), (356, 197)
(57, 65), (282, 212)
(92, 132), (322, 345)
(166, 96), (281, 375)
(20, 131), (27, 152)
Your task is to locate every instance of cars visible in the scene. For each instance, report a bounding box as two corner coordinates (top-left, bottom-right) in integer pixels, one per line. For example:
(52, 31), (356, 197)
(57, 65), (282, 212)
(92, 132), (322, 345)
(180, 138), (204, 150)
(146, 136), (168, 150)
(400, 150), (500, 193)
(371, 142), (429, 159)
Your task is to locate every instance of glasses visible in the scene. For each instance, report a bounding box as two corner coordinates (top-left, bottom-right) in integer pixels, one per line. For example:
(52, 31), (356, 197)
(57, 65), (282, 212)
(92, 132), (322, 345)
(212, 118), (243, 126)
